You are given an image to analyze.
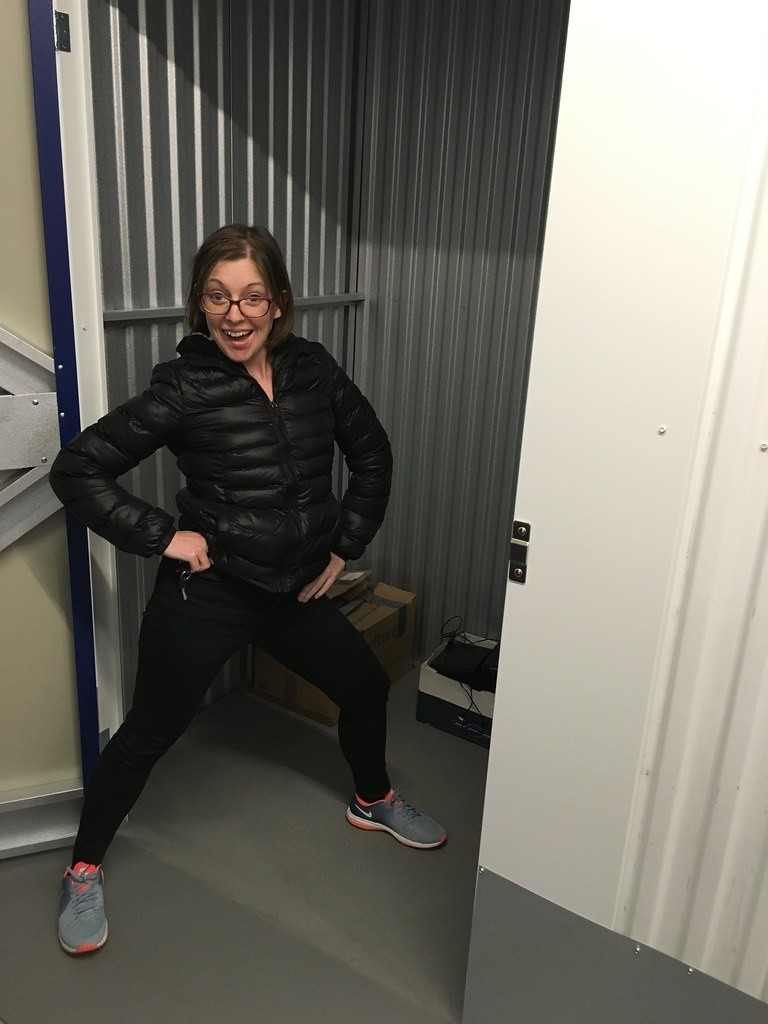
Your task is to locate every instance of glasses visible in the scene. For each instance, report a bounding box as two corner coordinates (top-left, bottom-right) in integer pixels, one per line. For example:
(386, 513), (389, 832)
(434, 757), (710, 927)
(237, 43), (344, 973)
(196, 292), (274, 319)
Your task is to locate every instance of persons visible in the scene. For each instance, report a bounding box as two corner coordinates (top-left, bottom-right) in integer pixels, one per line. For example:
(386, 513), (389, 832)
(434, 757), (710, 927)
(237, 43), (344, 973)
(48, 224), (453, 956)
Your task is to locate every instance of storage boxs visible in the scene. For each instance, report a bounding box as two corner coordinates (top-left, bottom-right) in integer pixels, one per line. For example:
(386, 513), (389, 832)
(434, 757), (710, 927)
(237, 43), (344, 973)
(416, 632), (500, 750)
(247, 569), (417, 727)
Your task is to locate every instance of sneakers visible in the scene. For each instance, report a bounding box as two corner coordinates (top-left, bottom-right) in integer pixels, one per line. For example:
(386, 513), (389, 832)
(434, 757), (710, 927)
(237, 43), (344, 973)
(55, 862), (110, 954)
(345, 787), (449, 851)
